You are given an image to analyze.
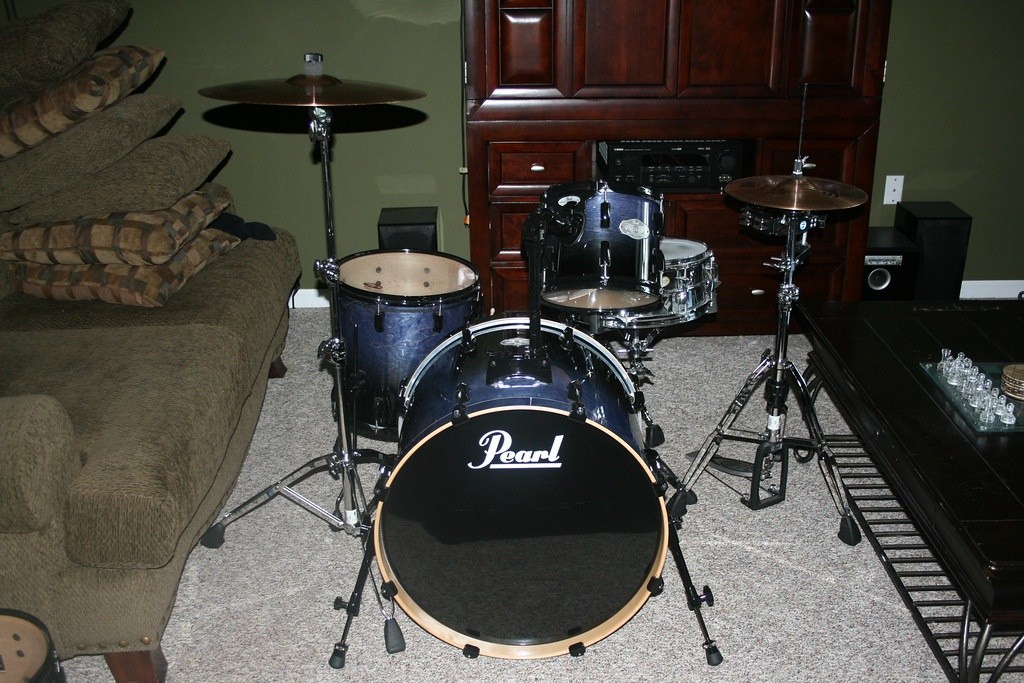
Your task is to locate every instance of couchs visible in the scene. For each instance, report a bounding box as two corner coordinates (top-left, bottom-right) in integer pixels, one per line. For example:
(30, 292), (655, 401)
(0, 226), (302, 683)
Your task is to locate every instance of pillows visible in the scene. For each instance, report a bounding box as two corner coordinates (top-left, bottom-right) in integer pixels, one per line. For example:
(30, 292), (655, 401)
(0, 191), (230, 263)
(0, 0), (133, 103)
(0, 41), (165, 158)
(12, 226), (244, 309)
(0, 93), (185, 214)
(7, 133), (232, 226)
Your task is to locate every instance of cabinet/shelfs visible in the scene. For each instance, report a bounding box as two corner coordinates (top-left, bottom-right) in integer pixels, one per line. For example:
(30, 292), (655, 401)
(462, 0), (893, 337)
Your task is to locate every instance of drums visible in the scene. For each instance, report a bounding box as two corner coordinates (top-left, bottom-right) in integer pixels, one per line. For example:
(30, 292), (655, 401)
(373, 318), (669, 659)
(540, 179), (664, 313)
(558, 236), (716, 329)
(330, 248), (486, 432)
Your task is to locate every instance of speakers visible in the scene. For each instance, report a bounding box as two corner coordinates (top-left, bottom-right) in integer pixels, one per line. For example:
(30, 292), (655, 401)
(861, 226), (916, 302)
(376, 206), (438, 250)
(894, 201), (973, 302)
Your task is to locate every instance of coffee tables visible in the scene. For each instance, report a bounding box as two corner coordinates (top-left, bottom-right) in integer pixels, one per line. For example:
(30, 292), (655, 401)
(795, 298), (1024, 683)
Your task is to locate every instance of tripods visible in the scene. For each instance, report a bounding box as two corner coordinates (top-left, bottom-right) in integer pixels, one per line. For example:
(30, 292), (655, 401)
(200, 53), (407, 653)
(665, 160), (862, 545)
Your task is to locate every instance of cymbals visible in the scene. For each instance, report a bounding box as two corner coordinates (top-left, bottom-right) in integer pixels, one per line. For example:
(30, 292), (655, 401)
(197, 74), (428, 107)
(724, 175), (869, 211)
